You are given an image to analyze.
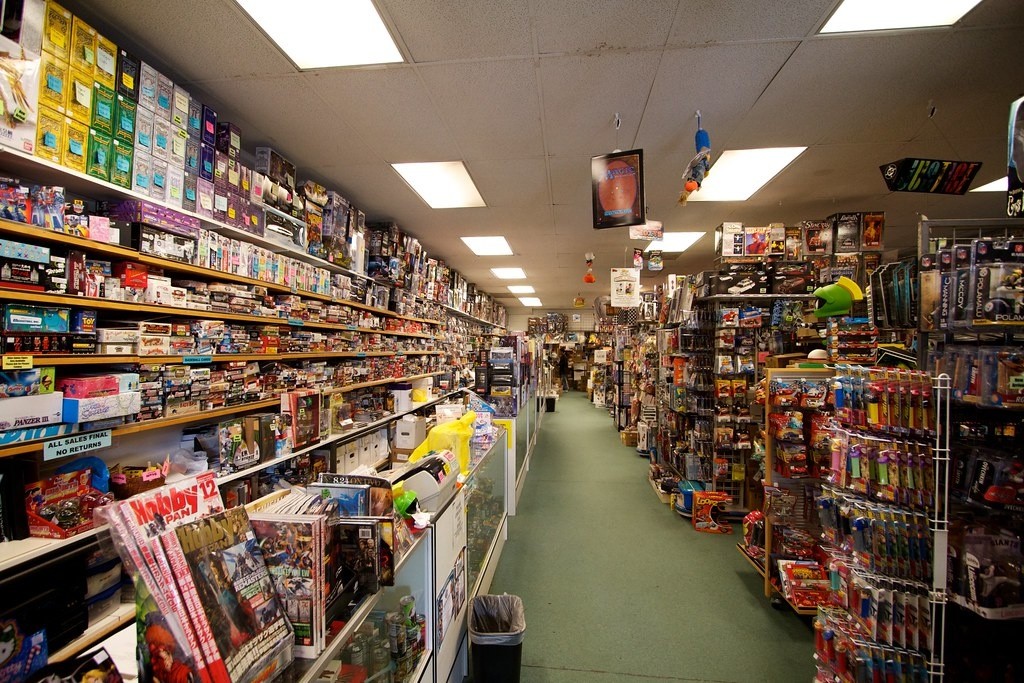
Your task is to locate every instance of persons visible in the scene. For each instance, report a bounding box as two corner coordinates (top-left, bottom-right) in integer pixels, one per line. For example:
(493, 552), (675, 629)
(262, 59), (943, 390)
(359, 539), (375, 563)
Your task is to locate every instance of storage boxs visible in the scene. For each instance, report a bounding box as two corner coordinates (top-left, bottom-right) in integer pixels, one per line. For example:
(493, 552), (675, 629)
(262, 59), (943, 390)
(280, 389), (321, 453)
(216, 121), (241, 162)
(255, 147), (296, 189)
(396, 417), (426, 449)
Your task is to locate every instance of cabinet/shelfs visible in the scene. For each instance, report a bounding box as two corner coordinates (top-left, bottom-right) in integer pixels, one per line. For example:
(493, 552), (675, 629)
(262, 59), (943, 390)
(0, 0), (1024, 683)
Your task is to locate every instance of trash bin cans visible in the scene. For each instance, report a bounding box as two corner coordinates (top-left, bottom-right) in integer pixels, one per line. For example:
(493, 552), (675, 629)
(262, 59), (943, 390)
(468, 594), (527, 683)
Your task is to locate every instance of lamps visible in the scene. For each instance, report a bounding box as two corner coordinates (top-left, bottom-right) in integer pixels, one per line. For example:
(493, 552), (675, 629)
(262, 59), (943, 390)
(572, 293), (584, 307)
(583, 252), (596, 283)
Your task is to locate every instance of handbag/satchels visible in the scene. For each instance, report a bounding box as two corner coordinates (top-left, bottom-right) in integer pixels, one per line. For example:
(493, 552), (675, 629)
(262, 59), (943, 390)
(407, 409), (477, 477)
